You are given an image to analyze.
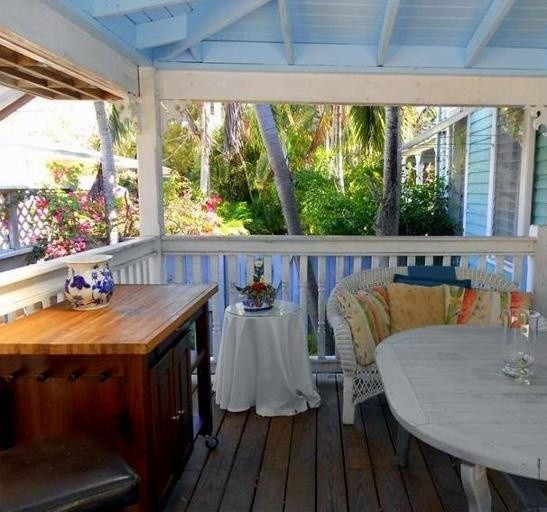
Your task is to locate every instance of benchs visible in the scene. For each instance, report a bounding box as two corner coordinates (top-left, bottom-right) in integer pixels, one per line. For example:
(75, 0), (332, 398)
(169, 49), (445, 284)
(323, 266), (541, 426)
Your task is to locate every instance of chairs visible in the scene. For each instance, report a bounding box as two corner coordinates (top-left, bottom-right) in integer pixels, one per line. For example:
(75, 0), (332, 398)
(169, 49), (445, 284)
(340, 266), (534, 367)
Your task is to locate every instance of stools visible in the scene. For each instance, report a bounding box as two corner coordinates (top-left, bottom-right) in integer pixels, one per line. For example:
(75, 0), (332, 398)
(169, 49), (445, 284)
(0, 419), (142, 511)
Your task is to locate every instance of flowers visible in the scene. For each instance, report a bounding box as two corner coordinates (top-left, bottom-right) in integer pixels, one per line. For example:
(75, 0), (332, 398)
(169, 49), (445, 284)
(234, 258), (283, 306)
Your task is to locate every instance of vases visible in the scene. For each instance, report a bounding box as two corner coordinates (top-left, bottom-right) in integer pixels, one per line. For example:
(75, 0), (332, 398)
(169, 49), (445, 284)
(242, 294), (274, 312)
(62, 255), (115, 312)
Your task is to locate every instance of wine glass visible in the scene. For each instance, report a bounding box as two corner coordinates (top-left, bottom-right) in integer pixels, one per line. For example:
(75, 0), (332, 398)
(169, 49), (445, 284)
(514, 356), (531, 387)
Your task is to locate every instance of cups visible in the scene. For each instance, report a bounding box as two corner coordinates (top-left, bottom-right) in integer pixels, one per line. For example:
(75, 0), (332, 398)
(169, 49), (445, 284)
(500, 309), (540, 379)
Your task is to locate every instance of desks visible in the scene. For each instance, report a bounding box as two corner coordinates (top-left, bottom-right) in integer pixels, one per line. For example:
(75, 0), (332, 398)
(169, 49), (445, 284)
(0, 283), (218, 507)
(221, 300), (305, 414)
(374, 325), (547, 512)
